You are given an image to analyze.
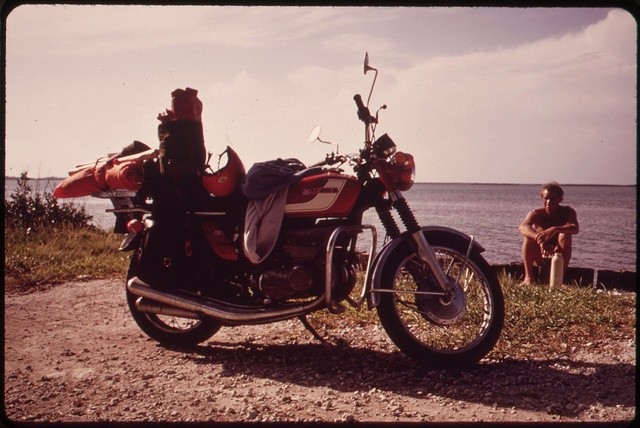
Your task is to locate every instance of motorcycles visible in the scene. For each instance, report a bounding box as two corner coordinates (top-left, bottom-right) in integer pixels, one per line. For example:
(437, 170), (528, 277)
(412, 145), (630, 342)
(89, 50), (506, 368)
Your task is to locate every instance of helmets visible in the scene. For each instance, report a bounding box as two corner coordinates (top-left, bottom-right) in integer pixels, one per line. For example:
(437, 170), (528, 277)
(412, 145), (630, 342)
(200, 147), (246, 199)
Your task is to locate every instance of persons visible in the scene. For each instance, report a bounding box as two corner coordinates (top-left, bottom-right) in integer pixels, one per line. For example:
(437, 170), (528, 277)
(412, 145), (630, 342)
(518, 181), (579, 288)
